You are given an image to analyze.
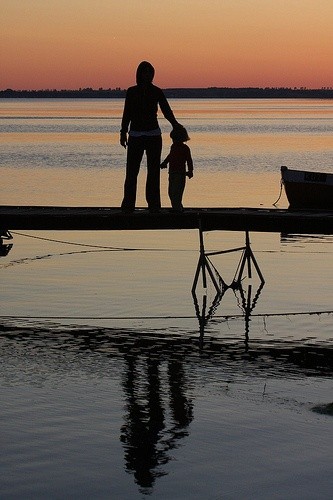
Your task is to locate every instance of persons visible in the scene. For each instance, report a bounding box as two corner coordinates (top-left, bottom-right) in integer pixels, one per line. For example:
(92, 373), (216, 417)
(120, 61), (184, 208)
(119, 352), (196, 494)
(160, 127), (194, 208)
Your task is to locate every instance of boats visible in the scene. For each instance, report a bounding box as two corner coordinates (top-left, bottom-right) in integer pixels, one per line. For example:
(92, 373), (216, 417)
(273, 166), (333, 207)
